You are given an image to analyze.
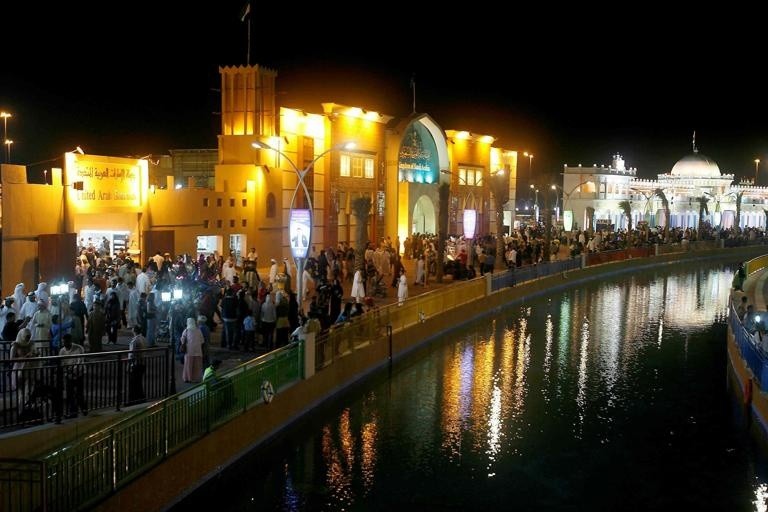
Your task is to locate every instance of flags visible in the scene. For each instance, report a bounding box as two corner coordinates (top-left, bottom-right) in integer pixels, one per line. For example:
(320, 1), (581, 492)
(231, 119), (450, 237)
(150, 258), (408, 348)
(241, 5), (250, 23)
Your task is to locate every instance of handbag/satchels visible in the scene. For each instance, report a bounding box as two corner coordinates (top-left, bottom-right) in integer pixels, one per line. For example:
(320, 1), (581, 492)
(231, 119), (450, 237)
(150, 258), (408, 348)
(179, 330), (187, 353)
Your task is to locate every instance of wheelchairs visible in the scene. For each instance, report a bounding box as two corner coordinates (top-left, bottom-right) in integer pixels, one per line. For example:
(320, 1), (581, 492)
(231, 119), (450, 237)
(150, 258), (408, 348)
(22, 362), (74, 423)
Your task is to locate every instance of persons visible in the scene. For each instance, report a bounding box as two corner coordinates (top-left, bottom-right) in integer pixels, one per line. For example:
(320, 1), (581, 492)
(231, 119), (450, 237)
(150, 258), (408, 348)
(2, 209), (500, 425)
(732, 270), (768, 358)
(502, 214), (768, 271)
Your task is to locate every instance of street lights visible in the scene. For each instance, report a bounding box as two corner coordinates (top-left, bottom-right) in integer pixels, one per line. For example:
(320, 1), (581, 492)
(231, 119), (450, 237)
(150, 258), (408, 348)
(629, 187), (671, 242)
(522, 151), (536, 192)
(549, 180), (594, 249)
(251, 138), (361, 381)
(160, 286), (185, 401)
(754, 159), (761, 186)
(704, 191), (736, 230)
(0, 111), (14, 139)
(50, 278), (69, 355)
(440, 168), (505, 271)
(5, 139), (14, 164)
